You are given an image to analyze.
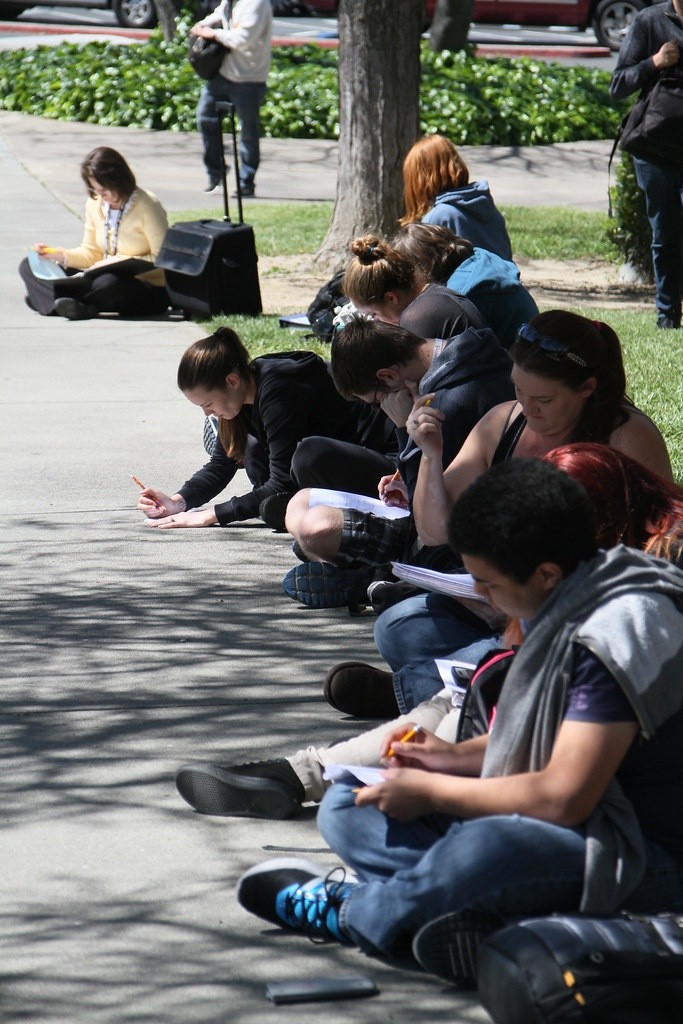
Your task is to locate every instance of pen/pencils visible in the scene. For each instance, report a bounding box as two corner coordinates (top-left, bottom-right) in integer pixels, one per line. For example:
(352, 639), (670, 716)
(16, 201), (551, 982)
(379, 724), (424, 769)
(27, 245), (57, 254)
(425, 400), (431, 406)
(130, 474), (166, 511)
(381, 469), (400, 501)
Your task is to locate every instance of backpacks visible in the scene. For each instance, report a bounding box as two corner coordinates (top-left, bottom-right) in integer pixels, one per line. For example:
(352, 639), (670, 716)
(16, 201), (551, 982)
(616, 75), (683, 175)
(307, 269), (350, 344)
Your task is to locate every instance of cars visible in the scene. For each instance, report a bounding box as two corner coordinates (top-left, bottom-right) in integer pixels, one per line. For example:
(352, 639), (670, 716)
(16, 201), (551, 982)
(422, 0), (654, 52)
(0, 0), (168, 30)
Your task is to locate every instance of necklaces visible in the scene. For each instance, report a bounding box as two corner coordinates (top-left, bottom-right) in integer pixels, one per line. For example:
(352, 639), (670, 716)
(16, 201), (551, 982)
(105, 201), (124, 258)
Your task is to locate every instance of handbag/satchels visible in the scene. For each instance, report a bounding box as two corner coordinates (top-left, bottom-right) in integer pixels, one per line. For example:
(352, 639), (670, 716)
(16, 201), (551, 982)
(188, 29), (229, 80)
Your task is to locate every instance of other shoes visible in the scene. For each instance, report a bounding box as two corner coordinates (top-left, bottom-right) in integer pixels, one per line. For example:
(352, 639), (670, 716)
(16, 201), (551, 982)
(206, 163), (231, 192)
(55, 296), (99, 320)
(284, 558), (394, 604)
(323, 663), (406, 719)
(477, 911), (683, 1024)
(230, 187), (255, 200)
(203, 415), (226, 455)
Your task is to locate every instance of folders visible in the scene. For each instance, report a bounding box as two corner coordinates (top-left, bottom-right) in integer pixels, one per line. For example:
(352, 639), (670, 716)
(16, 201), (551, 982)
(279, 313), (312, 328)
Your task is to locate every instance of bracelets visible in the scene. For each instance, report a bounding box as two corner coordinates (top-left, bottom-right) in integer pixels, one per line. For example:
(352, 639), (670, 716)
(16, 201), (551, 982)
(62, 251), (67, 267)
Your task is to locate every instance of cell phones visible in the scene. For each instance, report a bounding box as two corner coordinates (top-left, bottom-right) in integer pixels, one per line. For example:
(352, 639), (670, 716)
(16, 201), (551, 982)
(451, 666), (475, 687)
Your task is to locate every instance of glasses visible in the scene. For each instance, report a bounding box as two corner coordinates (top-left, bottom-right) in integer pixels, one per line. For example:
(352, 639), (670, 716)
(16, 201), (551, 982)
(368, 378), (387, 412)
(516, 325), (596, 376)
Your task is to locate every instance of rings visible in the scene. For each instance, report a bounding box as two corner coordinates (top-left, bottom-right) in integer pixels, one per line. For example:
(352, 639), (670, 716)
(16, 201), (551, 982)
(171, 517), (175, 522)
(414, 419), (419, 424)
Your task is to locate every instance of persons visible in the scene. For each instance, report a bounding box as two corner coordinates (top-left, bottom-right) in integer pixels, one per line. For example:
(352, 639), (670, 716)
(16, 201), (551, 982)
(138, 326), (370, 532)
(391, 224), (540, 350)
(177, 310), (683, 987)
(609, 0), (683, 328)
(397, 134), (512, 261)
(19, 146), (171, 320)
(342, 233), (489, 339)
(189, 0), (272, 199)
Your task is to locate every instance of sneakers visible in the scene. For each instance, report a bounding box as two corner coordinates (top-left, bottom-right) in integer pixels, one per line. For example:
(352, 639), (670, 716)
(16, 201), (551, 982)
(414, 908), (507, 987)
(235, 859), (366, 946)
(178, 756), (309, 820)
